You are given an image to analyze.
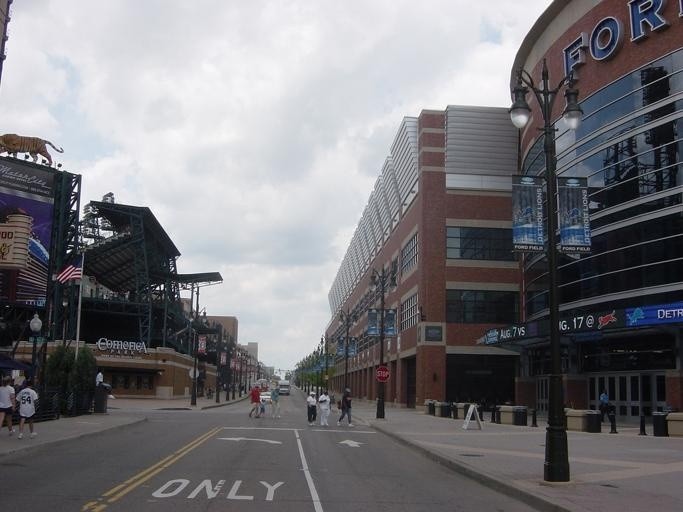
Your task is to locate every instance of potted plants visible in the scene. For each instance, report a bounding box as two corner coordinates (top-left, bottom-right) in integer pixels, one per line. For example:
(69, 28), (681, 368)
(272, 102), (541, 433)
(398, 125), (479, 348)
(65, 343), (99, 419)
(41, 337), (65, 416)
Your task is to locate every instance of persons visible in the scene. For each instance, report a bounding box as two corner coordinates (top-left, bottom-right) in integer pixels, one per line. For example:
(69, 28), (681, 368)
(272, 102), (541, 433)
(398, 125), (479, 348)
(599, 389), (611, 423)
(96, 366), (104, 387)
(0, 375), (17, 436)
(305, 386), (355, 427)
(15, 378), (39, 439)
(250, 384), (282, 420)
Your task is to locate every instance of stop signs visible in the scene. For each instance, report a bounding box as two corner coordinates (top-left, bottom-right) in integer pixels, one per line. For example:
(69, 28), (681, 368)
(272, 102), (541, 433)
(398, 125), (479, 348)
(375, 366), (390, 383)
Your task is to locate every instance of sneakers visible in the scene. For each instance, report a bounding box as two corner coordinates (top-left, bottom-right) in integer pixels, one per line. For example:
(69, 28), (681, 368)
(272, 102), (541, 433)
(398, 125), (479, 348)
(17, 432), (25, 439)
(29, 432), (38, 439)
(337, 421), (341, 427)
(348, 424), (355, 428)
(9, 428), (16, 436)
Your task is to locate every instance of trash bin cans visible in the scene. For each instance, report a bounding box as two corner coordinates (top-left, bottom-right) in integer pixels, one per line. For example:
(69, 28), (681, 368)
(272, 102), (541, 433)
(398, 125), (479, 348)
(652, 412), (669, 437)
(94, 382), (112, 413)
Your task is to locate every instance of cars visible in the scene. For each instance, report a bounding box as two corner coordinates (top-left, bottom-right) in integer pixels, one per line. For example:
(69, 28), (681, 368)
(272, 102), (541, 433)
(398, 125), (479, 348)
(252, 375), (291, 405)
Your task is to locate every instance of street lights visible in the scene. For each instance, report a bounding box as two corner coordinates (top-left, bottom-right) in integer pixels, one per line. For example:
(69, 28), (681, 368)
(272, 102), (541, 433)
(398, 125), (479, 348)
(507, 57), (592, 482)
(188, 287), (206, 405)
(335, 307), (359, 395)
(366, 263), (397, 419)
(29, 314), (42, 390)
(212, 323), (335, 404)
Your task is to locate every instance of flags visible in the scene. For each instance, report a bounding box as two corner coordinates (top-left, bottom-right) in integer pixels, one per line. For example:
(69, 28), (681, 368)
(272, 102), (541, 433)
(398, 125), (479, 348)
(56, 254), (83, 284)
